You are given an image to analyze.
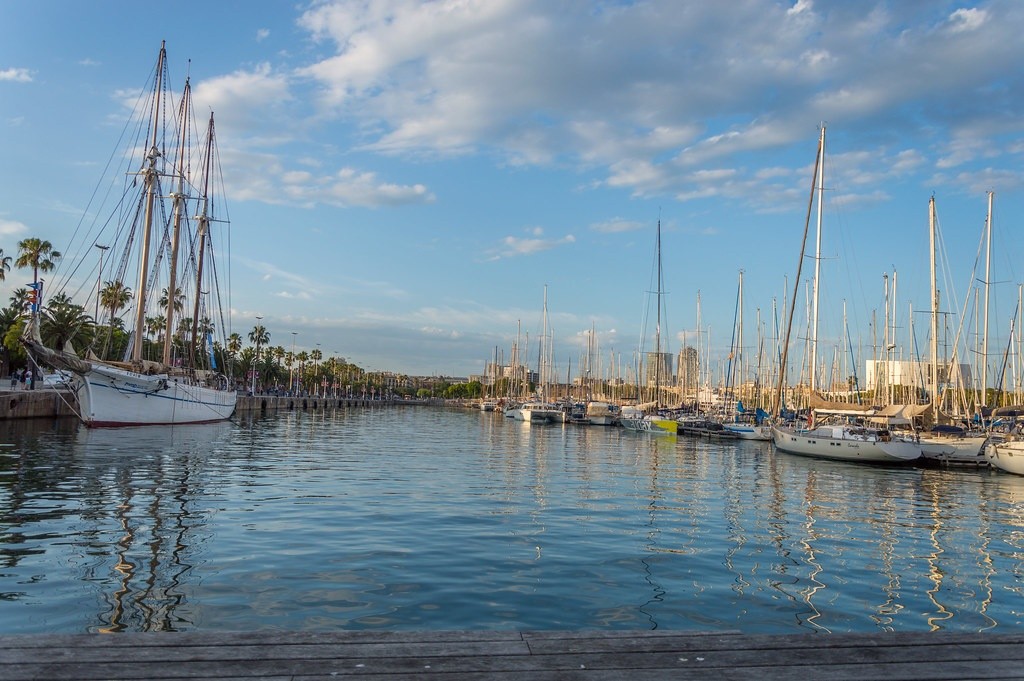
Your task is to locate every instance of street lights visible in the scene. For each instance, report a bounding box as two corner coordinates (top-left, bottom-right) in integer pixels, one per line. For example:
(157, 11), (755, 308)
(334, 351), (340, 396)
(290, 332), (298, 391)
(346, 357), (376, 400)
(93, 243), (112, 350)
(313, 343), (322, 395)
(251, 316), (264, 395)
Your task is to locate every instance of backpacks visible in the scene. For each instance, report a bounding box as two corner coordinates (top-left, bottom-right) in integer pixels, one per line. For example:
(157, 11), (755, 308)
(25, 372), (29, 378)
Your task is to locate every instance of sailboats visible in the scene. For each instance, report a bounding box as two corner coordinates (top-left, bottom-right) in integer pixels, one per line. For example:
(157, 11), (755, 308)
(475, 119), (1024, 478)
(16, 38), (240, 429)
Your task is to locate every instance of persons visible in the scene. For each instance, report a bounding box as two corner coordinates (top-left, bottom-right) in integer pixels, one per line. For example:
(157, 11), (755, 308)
(10, 364), (44, 390)
